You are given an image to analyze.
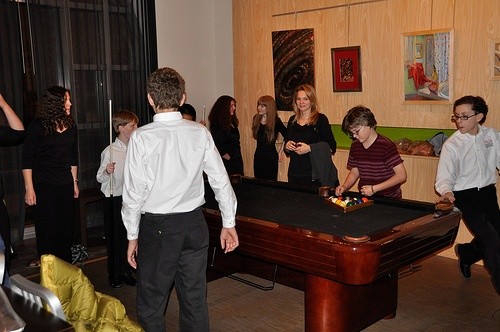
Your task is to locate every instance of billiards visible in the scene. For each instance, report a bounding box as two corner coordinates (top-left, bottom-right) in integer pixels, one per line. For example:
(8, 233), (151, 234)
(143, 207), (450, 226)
(329, 195), (369, 208)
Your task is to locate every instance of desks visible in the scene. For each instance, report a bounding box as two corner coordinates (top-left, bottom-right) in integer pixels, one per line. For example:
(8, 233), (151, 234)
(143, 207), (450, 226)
(200, 174), (464, 332)
(276, 142), (500, 265)
(1, 285), (76, 332)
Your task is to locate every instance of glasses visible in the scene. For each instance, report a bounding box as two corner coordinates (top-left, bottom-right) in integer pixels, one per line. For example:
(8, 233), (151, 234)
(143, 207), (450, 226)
(451, 114), (477, 120)
(351, 127), (363, 136)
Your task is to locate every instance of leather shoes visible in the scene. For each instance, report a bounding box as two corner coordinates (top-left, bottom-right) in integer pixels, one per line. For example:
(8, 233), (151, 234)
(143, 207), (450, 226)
(110, 277), (122, 289)
(457, 243), (471, 278)
(122, 274), (135, 286)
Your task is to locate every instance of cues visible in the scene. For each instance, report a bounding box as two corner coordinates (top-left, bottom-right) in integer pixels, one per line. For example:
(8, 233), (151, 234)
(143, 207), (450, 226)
(108, 100), (114, 285)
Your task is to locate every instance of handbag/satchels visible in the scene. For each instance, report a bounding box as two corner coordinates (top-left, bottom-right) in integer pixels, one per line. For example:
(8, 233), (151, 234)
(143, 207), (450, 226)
(71, 243), (88, 265)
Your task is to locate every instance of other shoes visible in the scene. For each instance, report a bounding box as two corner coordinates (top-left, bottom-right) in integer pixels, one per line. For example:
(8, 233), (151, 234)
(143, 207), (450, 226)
(25, 258), (41, 268)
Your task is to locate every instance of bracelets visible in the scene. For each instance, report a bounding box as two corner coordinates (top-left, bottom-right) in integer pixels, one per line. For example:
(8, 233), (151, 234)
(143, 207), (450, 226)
(371, 185), (375, 194)
(74, 179), (79, 182)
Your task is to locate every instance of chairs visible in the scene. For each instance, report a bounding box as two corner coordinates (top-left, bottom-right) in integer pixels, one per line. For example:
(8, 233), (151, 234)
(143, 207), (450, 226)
(10, 273), (67, 320)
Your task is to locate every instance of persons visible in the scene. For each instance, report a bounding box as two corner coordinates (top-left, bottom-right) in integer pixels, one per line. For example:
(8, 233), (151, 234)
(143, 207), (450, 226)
(335, 104), (407, 199)
(96, 110), (139, 288)
(177, 103), (196, 121)
(208, 95), (244, 176)
(252, 95), (286, 181)
(0, 94), (26, 276)
(434, 95), (500, 295)
(21, 86), (80, 263)
(283, 84), (340, 187)
(121, 67), (239, 332)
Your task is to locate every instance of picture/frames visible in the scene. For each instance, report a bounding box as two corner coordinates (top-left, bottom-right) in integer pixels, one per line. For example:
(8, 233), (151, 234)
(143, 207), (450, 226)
(401, 28), (457, 105)
(331, 45), (363, 92)
(489, 38), (500, 81)
